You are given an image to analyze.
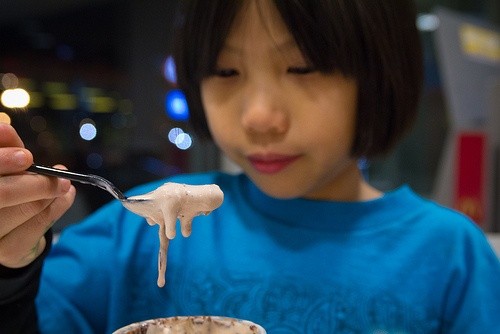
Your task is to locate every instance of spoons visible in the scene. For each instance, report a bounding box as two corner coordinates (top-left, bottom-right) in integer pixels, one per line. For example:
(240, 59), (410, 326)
(25, 162), (224, 221)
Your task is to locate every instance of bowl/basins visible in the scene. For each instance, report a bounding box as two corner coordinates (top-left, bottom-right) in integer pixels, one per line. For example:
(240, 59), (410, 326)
(111, 316), (266, 334)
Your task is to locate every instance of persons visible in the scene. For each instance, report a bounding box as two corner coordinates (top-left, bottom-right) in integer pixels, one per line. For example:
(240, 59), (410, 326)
(1, 0), (500, 334)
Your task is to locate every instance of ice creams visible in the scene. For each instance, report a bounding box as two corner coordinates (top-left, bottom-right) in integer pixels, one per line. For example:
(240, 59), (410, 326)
(126, 180), (224, 287)
